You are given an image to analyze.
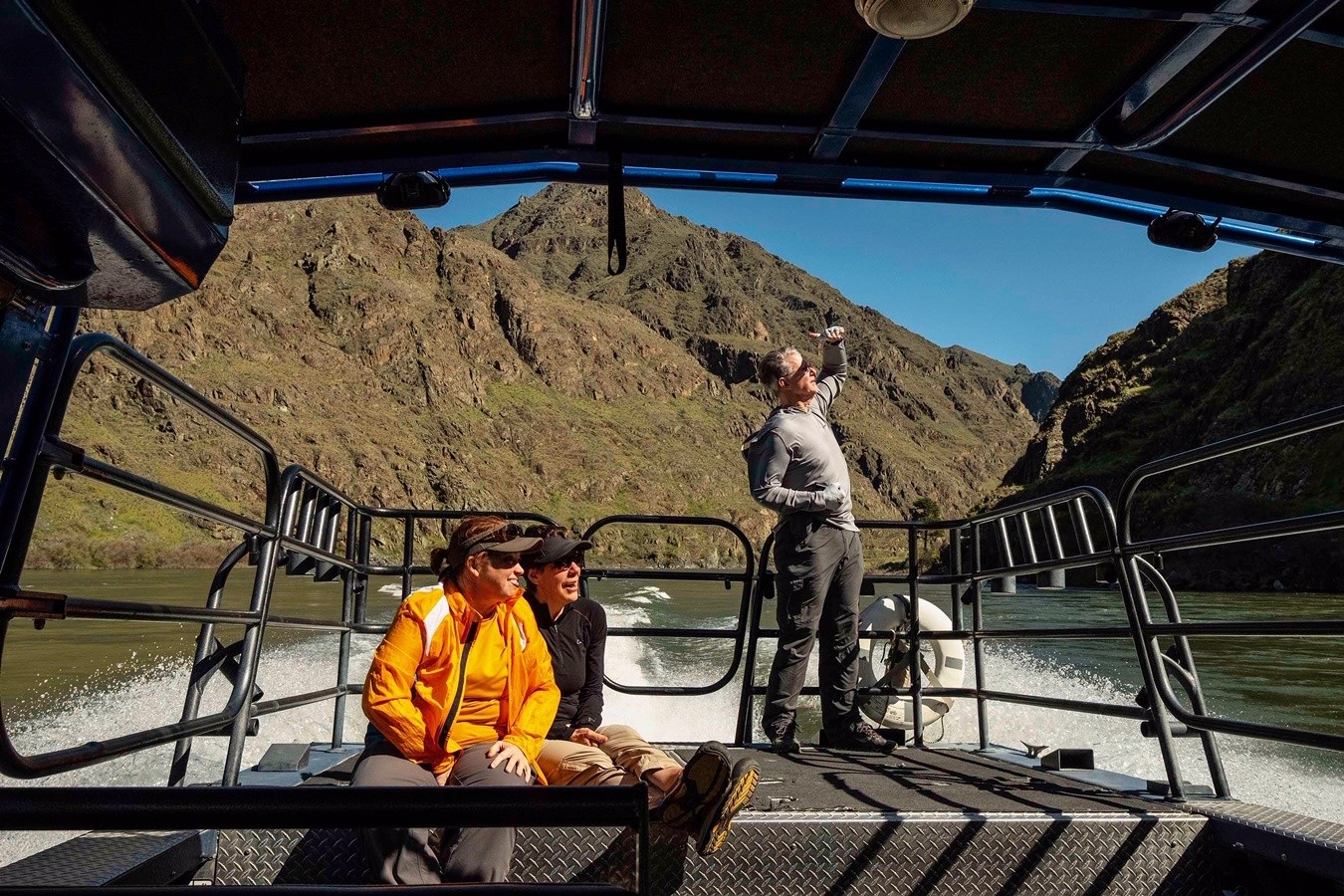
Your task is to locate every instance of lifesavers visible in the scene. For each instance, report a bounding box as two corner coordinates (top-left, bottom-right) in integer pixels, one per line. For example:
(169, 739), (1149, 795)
(858, 593), (966, 730)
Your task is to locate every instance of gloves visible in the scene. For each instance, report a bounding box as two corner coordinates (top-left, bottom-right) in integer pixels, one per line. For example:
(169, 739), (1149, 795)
(823, 483), (846, 512)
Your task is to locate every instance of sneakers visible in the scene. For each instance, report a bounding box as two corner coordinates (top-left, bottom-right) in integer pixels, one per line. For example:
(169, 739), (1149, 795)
(826, 721), (899, 752)
(767, 718), (803, 753)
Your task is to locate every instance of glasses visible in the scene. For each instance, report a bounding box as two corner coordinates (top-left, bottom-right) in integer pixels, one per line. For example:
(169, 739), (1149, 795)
(779, 359), (810, 378)
(544, 549), (584, 570)
(463, 523), (524, 553)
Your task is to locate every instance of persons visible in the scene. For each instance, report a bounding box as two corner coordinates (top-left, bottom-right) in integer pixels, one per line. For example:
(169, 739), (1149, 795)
(349, 514), (561, 885)
(740, 326), (899, 754)
(519, 524), (761, 859)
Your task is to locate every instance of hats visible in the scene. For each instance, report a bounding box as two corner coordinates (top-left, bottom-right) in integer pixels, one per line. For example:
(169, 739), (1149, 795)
(521, 536), (593, 564)
(446, 522), (544, 557)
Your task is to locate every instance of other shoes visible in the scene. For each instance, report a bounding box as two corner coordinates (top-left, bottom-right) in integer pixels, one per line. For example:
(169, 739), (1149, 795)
(657, 741), (732, 830)
(696, 758), (761, 857)
